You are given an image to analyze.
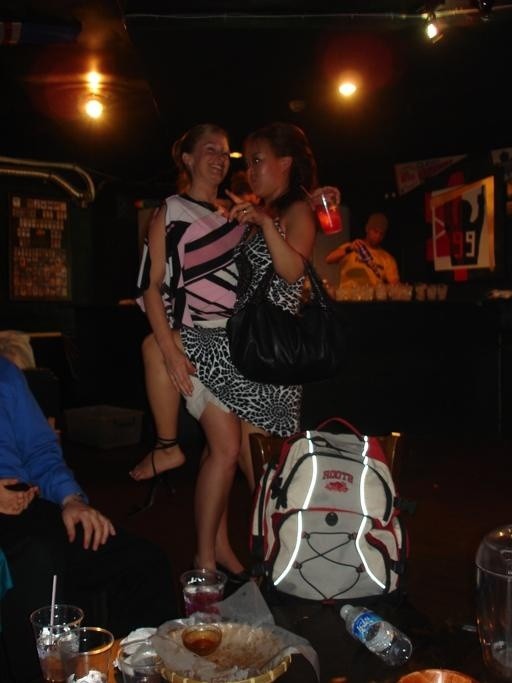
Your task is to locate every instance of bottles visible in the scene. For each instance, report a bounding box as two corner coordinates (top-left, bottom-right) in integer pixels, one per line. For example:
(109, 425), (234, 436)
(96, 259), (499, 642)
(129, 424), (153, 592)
(356, 241), (384, 277)
(338, 604), (413, 668)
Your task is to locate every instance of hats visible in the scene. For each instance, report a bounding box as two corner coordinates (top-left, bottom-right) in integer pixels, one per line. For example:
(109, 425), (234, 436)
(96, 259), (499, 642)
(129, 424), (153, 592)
(368, 214), (388, 231)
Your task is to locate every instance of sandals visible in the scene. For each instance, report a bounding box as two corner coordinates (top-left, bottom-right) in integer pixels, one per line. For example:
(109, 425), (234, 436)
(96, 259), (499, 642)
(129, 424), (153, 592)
(191, 557), (251, 590)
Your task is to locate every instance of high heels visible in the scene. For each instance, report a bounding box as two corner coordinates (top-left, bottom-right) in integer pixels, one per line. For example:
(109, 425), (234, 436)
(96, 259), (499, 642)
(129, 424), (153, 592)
(126, 435), (183, 519)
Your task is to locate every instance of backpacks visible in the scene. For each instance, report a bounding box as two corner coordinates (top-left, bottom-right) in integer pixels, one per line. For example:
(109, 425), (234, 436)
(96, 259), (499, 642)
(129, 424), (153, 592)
(255, 415), (401, 596)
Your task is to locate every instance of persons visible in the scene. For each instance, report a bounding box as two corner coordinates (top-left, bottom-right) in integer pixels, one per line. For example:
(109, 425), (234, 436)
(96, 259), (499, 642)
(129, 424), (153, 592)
(322, 203), (398, 284)
(0, 352), (177, 642)
(125, 123), (322, 489)
(152, 123), (269, 583)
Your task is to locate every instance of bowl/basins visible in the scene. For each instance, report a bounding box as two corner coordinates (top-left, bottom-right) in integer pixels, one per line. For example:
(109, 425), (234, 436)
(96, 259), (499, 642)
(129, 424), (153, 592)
(398, 668), (476, 683)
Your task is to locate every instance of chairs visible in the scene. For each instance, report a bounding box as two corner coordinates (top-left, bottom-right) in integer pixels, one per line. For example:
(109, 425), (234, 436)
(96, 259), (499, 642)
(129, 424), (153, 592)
(249, 431), (402, 490)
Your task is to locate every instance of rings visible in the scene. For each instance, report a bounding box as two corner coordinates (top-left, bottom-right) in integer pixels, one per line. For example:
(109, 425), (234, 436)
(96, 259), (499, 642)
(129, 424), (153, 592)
(242, 208), (247, 215)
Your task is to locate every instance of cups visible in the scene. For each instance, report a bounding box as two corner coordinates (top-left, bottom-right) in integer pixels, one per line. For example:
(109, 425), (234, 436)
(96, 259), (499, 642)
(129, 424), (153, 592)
(311, 189), (345, 237)
(31, 568), (227, 682)
(321, 280), (450, 302)
(474, 526), (512, 681)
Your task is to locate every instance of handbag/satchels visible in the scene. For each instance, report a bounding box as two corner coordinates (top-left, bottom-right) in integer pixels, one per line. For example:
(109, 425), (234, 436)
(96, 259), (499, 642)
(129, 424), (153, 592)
(224, 253), (335, 382)
